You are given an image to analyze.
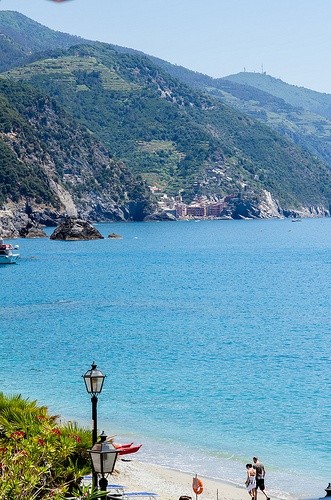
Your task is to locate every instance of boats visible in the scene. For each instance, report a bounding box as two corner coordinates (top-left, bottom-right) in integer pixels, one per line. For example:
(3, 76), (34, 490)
(0, 244), (20, 264)
(112, 441), (143, 455)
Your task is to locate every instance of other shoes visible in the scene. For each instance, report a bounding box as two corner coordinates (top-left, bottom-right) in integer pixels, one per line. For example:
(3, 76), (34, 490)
(252, 496), (255, 500)
(266, 497), (270, 500)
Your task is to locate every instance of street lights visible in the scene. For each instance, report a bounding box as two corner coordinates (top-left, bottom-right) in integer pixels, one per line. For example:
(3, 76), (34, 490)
(85, 430), (121, 500)
(81, 360), (108, 500)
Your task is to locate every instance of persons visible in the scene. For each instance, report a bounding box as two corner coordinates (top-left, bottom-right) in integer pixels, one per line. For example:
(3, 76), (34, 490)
(244, 463), (257, 500)
(253, 456), (270, 500)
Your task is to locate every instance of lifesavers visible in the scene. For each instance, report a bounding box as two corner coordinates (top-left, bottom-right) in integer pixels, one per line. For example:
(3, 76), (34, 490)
(192, 477), (203, 494)
